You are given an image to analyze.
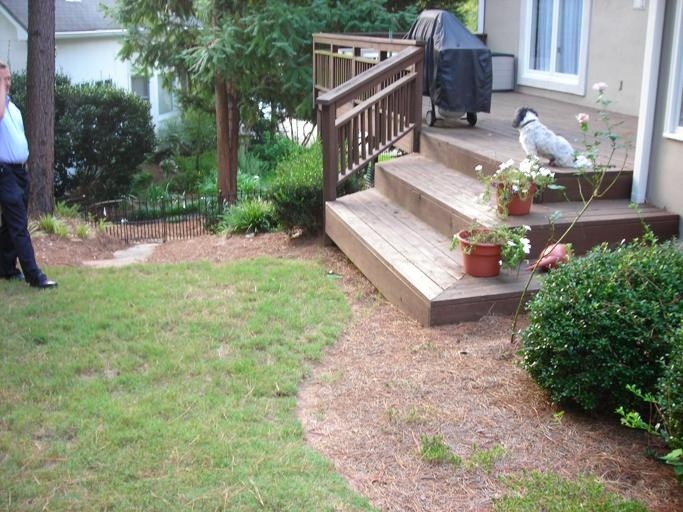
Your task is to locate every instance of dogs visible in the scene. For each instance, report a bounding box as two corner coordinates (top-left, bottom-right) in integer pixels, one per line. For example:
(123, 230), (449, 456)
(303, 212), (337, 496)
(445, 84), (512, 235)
(511, 105), (591, 169)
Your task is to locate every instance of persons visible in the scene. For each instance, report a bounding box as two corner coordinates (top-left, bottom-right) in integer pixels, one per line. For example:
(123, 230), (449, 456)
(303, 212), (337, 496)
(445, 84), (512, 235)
(0, 62), (56, 287)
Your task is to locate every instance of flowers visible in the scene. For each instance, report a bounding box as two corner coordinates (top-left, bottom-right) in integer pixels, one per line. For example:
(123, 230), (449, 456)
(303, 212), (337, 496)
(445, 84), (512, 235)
(475, 154), (557, 220)
(450, 224), (533, 272)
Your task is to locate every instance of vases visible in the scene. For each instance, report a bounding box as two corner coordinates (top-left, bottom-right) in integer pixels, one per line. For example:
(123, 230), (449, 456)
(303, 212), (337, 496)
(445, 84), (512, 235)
(457, 228), (502, 277)
(491, 181), (536, 216)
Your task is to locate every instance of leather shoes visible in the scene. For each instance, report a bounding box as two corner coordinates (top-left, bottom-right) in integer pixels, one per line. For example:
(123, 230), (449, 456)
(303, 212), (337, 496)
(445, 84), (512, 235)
(40, 280), (58, 289)
(16, 274), (25, 281)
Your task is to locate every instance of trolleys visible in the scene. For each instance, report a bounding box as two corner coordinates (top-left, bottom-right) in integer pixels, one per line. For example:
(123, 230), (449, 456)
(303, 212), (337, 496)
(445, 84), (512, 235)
(408, 9), (492, 129)
